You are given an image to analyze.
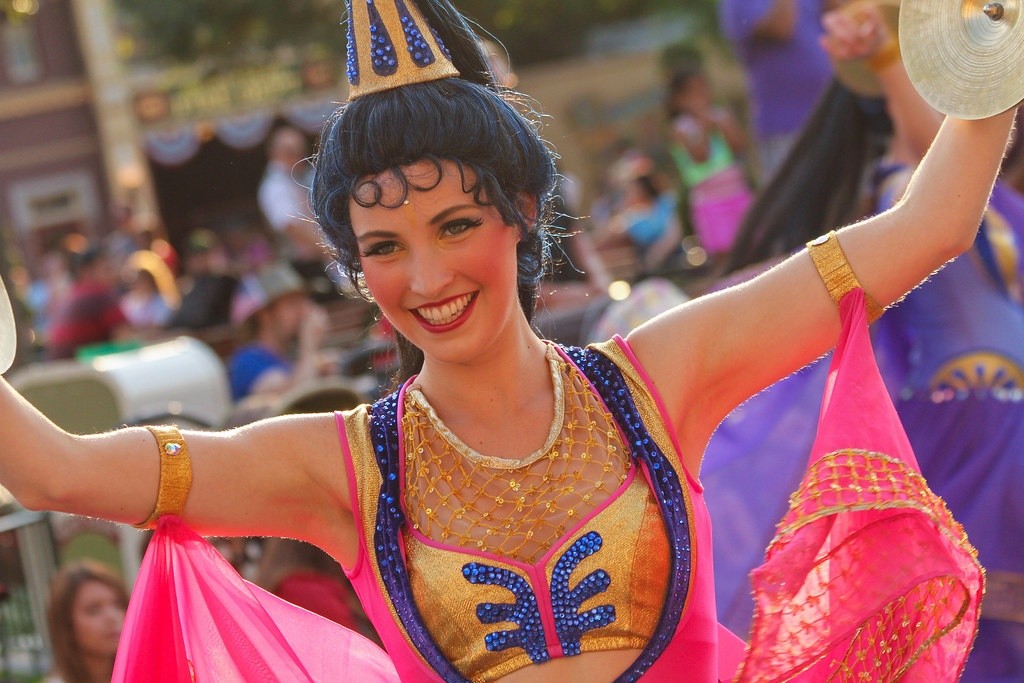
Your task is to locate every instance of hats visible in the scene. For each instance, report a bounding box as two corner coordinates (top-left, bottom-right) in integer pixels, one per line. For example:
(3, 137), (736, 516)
(344, 0), (460, 101)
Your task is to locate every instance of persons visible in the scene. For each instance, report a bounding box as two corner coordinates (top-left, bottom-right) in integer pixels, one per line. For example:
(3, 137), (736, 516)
(46, 561), (132, 682)
(699, 3), (1024, 680)
(43, 0), (829, 640)
(0, 0), (1024, 682)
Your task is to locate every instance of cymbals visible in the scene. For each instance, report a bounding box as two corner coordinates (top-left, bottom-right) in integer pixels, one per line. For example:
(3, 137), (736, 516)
(898, 1), (1024, 120)
(0, 274), (18, 376)
